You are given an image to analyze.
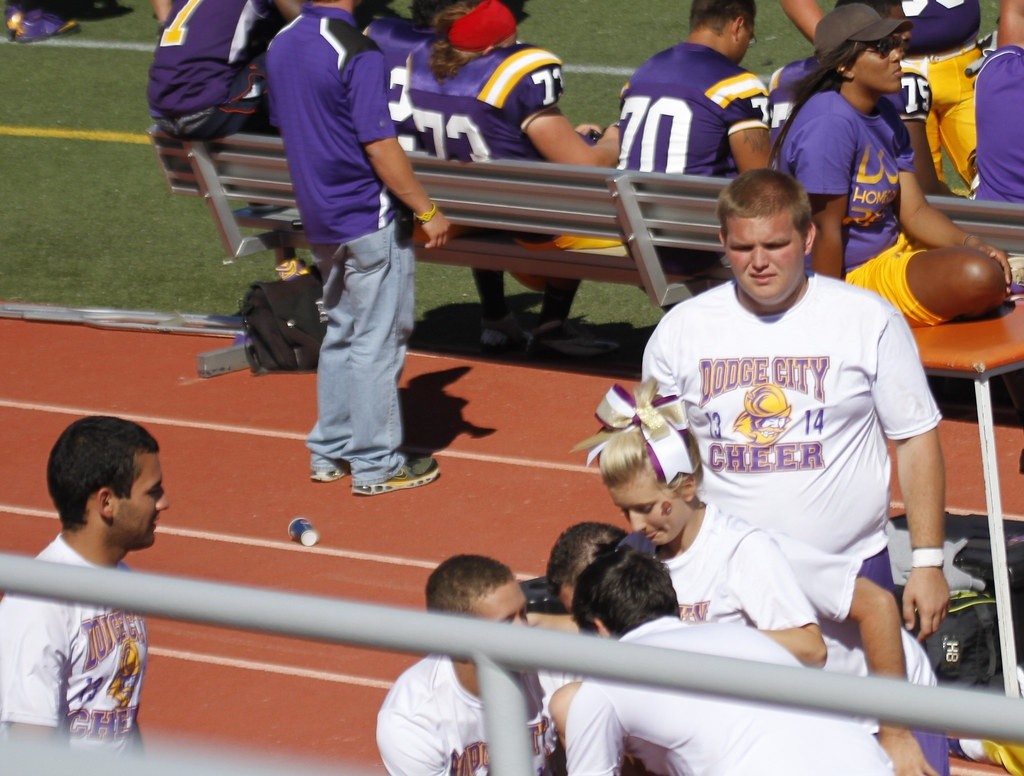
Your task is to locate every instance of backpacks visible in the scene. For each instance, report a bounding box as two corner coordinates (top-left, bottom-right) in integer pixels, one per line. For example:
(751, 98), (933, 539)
(908, 591), (1006, 696)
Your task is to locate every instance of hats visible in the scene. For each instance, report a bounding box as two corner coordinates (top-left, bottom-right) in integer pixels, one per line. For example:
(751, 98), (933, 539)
(813, 3), (913, 65)
(448, 0), (516, 52)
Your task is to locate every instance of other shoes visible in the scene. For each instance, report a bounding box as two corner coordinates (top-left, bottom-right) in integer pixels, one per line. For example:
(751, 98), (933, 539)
(274, 256), (322, 283)
(3, 7), (81, 44)
(1007, 256), (1024, 286)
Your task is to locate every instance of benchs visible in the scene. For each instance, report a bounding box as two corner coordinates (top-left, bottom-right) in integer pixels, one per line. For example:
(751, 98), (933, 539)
(145, 127), (1024, 321)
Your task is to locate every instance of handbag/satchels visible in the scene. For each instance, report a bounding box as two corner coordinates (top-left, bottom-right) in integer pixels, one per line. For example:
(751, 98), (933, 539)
(239, 265), (327, 374)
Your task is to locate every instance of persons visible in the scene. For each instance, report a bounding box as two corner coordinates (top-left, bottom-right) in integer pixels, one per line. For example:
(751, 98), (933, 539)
(374, 553), (580, 776)
(151, 1), (460, 226)
(618, 0), (770, 178)
(779, 0), (982, 200)
(0, 412), (168, 746)
(764, 4), (1012, 323)
(405, 1), (618, 347)
(969, 0), (1024, 205)
(572, 377), (829, 668)
(565, 546), (949, 775)
(642, 168), (953, 640)
(547, 522), (631, 745)
(264, 1), (450, 497)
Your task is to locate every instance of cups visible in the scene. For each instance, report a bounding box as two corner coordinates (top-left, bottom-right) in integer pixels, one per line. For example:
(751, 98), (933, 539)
(289, 517), (318, 546)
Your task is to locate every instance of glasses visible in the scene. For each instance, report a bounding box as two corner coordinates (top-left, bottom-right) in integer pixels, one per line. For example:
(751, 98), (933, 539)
(865, 35), (901, 58)
(734, 14), (757, 48)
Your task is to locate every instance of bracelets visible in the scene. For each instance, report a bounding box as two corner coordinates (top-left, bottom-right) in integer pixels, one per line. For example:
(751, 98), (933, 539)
(963, 234), (976, 249)
(910, 550), (944, 567)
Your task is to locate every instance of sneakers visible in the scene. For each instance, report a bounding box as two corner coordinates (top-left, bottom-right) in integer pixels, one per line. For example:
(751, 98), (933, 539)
(479, 309), (618, 357)
(310, 460), (350, 482)
(352, 457), (438, 495)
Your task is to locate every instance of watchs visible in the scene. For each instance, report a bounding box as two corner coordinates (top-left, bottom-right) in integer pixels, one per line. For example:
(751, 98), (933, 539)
(414, 201), (436, 223)
